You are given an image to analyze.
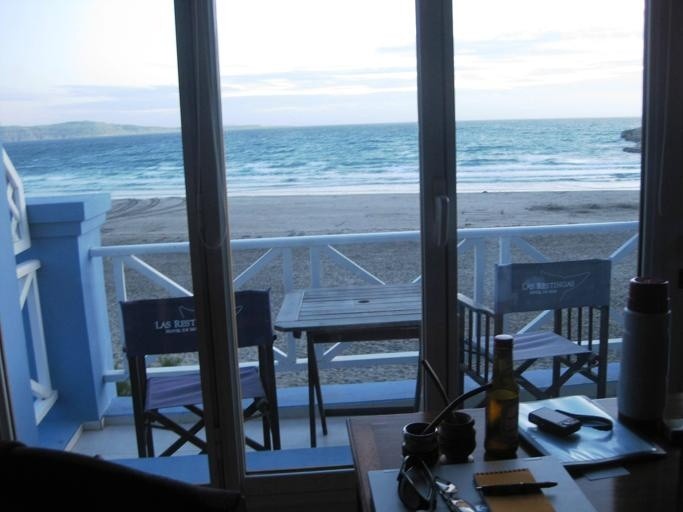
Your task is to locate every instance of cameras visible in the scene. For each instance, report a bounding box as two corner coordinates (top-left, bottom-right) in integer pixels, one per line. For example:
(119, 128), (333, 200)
(529, 406), (581, 438)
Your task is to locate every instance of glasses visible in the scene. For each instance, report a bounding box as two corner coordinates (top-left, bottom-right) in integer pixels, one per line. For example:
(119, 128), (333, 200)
(397, 455), (437, 511)
(434, 475), (477, 512)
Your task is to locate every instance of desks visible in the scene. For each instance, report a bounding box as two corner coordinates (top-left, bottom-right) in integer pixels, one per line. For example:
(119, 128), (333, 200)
(343, 387), (681, 511)
(272, 283), (425, 449)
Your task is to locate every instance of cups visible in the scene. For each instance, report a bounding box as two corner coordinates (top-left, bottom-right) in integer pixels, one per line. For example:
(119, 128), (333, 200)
(438, 411), (477, 461)
(402, 421), (440, 468)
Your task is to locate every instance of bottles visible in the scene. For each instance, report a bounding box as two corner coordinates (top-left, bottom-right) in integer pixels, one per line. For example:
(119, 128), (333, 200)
(483, 333), (519, 453)
(616, 274), (674, 423)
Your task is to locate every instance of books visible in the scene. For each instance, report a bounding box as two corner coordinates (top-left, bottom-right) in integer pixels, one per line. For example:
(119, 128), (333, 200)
(517, 394), (657, 466)
(368, 455), (598, 512)
(472, 469), (557, 512)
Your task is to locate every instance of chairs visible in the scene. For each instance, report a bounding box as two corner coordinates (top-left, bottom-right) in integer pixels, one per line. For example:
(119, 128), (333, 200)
(114, 285), (281, 461)
(452, 253), (613, 409)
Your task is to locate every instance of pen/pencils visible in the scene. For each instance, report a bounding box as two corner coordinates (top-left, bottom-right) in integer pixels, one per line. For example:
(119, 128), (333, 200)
(476, 482), (558, 493)
